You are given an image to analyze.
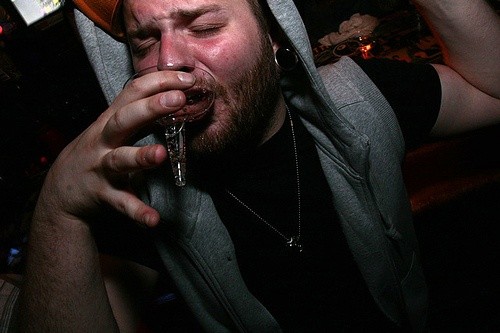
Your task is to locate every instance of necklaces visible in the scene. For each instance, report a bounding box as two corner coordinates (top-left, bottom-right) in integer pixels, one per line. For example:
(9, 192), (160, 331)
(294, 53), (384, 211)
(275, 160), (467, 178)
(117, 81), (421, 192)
(200, 103), (304, 254)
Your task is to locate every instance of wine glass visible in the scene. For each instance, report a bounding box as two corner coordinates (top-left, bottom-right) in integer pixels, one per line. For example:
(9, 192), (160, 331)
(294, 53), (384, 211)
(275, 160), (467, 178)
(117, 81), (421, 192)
(121, 64), (217, 188)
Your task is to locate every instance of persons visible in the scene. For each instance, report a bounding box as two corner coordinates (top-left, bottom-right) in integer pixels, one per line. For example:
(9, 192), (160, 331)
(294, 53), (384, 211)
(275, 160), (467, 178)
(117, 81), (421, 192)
(17, 0), (500, 333)
